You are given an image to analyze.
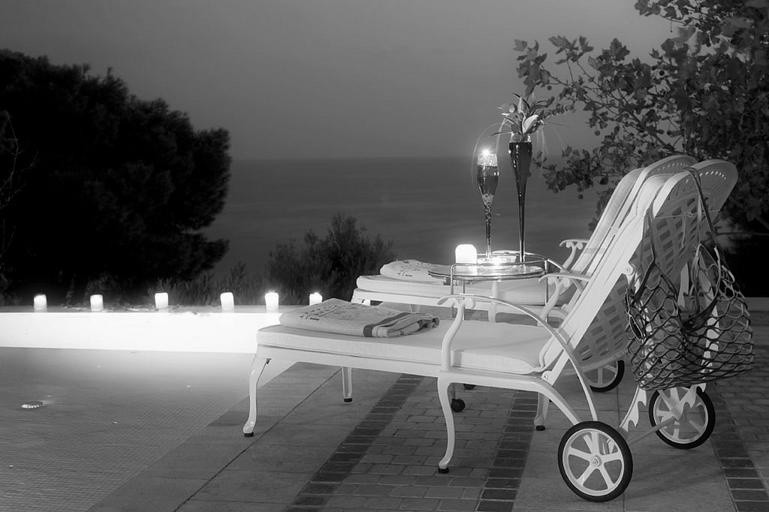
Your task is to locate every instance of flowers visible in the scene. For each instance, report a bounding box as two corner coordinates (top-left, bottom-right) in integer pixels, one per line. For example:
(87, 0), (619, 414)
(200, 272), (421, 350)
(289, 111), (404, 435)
(471, 89), (546, 177)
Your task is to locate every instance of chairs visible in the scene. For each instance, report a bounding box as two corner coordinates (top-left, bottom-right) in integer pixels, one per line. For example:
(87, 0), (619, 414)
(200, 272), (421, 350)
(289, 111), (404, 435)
(241, 158), (738, 503)
(341, 155), (700, 412)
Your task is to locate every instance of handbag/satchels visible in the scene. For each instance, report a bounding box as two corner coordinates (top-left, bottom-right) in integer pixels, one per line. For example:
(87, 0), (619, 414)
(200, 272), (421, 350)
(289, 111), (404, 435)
(622, 163), (756, 390)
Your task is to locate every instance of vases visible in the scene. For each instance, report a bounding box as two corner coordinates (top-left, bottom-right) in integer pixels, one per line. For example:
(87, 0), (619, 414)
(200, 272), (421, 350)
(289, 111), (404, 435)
(507, 142), (532, 268)
